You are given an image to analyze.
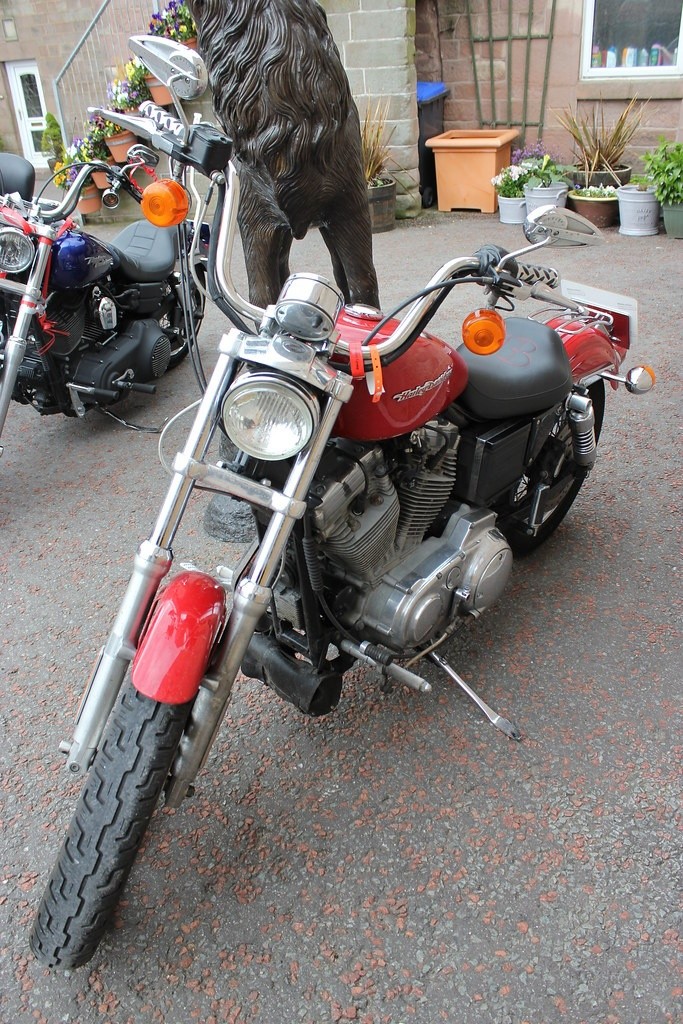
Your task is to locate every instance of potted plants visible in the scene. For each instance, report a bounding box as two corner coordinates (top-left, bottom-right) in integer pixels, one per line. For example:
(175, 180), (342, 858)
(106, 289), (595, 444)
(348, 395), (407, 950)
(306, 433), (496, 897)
(638, 133), (683, 239)
(41, 112), (64, 175)
(359, 86), (422, 233)
(616, 173), (661, 236)
(547, 88), (667, 189)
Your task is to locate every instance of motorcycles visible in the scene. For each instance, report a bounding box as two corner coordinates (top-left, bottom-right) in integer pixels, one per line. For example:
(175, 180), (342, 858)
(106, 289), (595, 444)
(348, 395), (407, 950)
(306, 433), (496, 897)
(29, 36), (655, 969)
(2, 146), (211, 462)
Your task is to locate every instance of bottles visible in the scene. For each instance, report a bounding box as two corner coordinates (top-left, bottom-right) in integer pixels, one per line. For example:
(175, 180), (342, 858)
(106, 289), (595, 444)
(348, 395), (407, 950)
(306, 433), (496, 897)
(591, 46), (663, 68)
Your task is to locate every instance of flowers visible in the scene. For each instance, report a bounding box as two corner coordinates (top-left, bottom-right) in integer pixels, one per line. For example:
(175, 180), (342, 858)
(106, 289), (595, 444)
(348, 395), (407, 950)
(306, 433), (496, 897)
(491, 140), (577, 198)
(51, 0), (205, 193)
(574, 182), (617, 197)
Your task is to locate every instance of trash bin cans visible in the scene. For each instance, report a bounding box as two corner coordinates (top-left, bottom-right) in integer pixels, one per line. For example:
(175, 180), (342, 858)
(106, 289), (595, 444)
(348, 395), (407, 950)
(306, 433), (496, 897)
(416, 81), (451, 208)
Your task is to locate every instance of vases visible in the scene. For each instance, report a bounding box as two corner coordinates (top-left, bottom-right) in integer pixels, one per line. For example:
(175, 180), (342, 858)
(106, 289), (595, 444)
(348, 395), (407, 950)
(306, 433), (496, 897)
(567, 190), (620, 228)
(425, 129), (521, 214)
(498, 194), (527, 224)
(523, 181), (569, 215)
(75, 38), (197, 214)
